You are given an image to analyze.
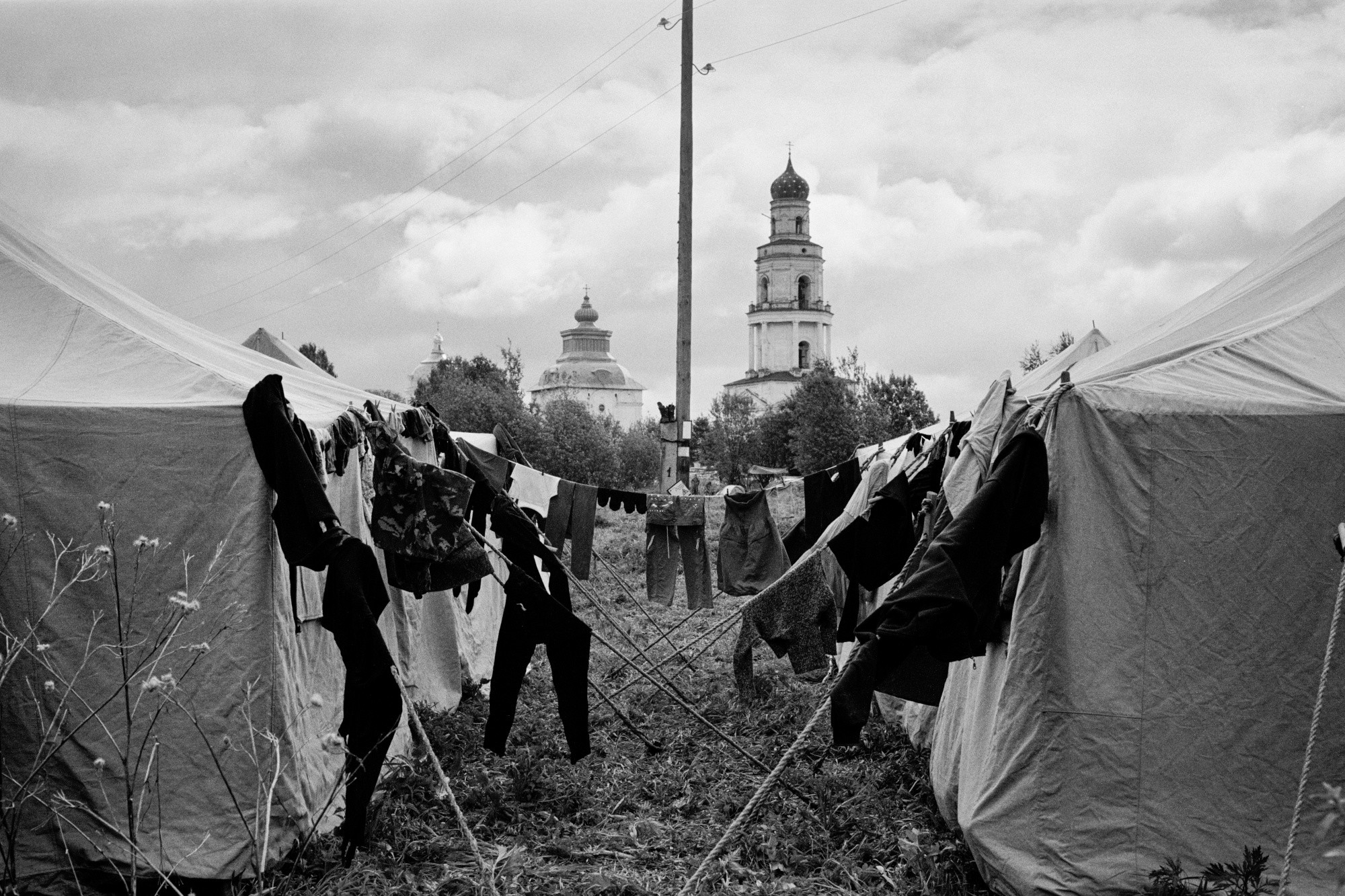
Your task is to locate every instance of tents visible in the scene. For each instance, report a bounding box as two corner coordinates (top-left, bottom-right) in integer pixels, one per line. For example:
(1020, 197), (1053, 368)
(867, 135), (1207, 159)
(832, 191), (1345, 896)
(0, 206), (510, 884)
